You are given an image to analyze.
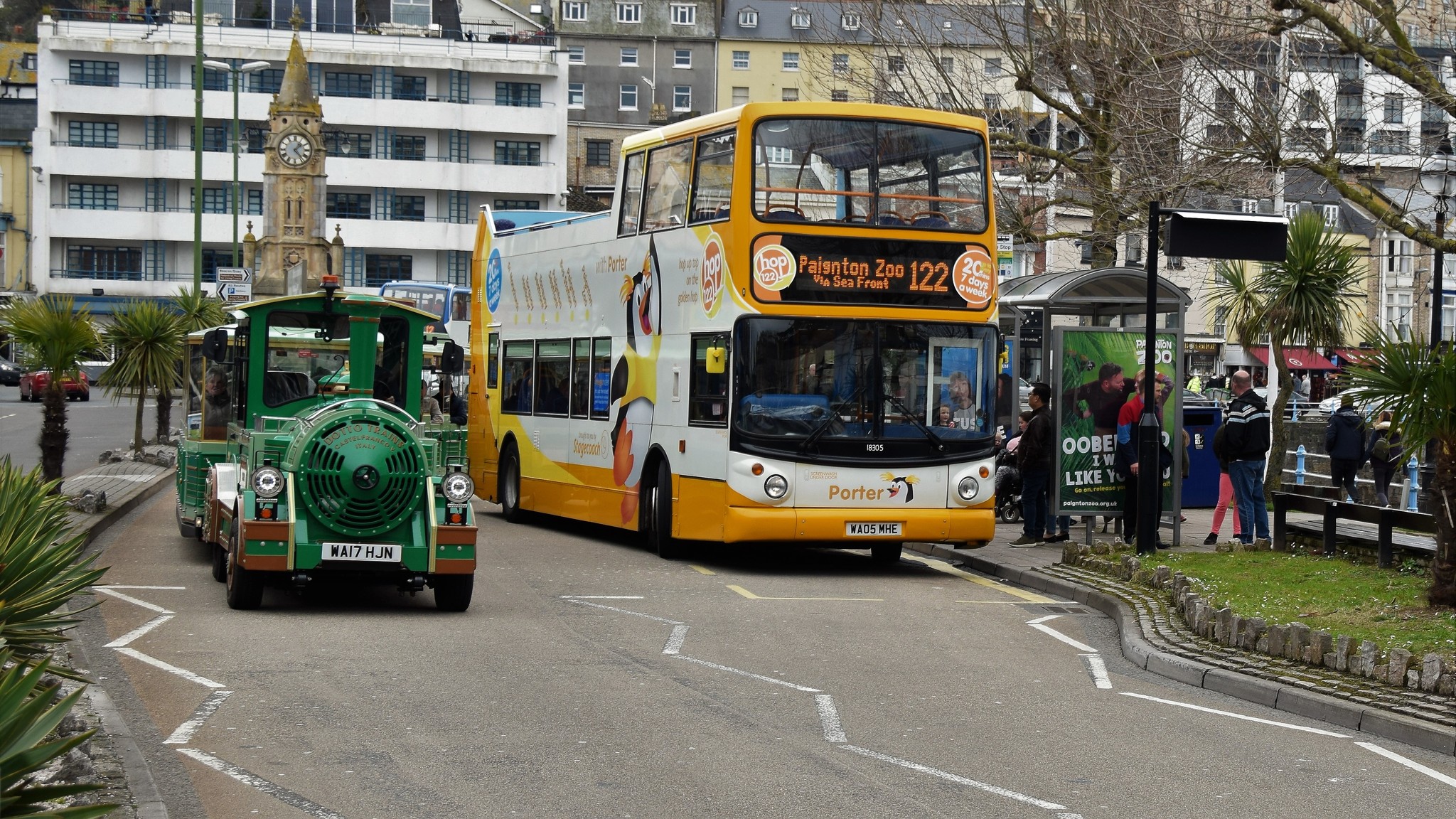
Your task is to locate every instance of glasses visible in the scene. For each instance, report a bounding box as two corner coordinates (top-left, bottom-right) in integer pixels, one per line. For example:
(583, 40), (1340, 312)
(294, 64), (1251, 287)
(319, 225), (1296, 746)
(1028, 393), (1034, 397)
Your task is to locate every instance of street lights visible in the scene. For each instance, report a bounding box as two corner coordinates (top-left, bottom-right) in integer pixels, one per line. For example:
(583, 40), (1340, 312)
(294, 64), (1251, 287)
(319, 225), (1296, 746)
(204, 60), (271, 267)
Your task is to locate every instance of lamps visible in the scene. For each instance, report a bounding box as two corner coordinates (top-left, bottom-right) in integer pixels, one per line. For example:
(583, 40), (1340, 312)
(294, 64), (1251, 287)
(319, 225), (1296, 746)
(201, 290), (209, 299)
(32, 166), (43, 182)
(559, 189), (570, 206)
(92, 287), (104, 296)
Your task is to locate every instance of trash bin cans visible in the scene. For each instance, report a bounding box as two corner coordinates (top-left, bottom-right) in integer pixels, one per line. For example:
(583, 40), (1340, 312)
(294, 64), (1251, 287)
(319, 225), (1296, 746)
(1181, 405), (1224, 510)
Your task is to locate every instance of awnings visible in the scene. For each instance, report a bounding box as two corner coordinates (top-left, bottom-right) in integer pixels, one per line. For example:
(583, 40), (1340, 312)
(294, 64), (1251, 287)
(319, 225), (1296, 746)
(1331, 348), (1383, 367)
(1248, 346), (1338, 370)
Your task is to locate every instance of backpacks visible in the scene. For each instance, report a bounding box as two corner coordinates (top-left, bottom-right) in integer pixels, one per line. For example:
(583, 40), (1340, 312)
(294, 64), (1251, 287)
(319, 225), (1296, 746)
(1371, 430), (1396, 460)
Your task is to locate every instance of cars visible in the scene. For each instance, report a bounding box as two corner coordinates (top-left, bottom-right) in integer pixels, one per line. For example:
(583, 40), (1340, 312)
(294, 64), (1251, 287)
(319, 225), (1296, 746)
(1251, 387), (1311, 419)
(1019, 377), (1035, 403)
(1318, 386), (1404, 422)
(19, 361), (90, 402)
(1183, 388), (1211, 407)
(0, 355), (29, 383)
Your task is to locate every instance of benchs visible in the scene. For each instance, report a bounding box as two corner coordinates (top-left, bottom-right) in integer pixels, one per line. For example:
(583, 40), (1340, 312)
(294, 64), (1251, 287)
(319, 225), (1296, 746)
(1270, 490), (1434, 568)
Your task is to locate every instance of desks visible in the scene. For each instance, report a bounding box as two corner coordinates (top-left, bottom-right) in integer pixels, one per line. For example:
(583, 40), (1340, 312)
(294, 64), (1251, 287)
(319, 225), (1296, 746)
(489, 35), (511, 42)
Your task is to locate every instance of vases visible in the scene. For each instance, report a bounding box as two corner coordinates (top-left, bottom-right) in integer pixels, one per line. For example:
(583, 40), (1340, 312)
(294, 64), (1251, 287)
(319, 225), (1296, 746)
(138, 8), (144, 13)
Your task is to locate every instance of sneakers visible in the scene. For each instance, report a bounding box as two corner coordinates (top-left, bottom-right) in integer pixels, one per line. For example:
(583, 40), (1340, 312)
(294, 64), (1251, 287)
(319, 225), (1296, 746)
(1157, 543), (1170, 549)
(1385, 504), (1391, 509)
(1180, 516), (1187, 522)
(1232, 533), (1240, 538)
(1035, 537), (1045, 546)
(1070, 518), (1077, 524)
(1007, 532), (1036, 548)
(1203, 533), (1218, 545)
(1081, 516), (1087, 523)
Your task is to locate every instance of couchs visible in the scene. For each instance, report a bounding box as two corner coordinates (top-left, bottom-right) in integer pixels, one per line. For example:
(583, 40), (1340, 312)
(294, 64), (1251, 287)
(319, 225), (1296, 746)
(169, 11), (222, 26)
(378, 22), (442, 37)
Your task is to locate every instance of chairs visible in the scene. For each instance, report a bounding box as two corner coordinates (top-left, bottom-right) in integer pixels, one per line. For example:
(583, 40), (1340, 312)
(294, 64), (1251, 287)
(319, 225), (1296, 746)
(263, 372), (308, 408)
(692, 204), (951, 229)
(520, 366), (562, 413)
(696, 366), (721, 419)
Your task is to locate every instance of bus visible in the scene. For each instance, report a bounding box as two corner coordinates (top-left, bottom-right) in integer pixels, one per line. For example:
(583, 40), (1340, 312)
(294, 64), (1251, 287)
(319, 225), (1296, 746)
(375, 280), (472, 349)
(171, 273), (480, 615)
(467, 98), (1008, 563)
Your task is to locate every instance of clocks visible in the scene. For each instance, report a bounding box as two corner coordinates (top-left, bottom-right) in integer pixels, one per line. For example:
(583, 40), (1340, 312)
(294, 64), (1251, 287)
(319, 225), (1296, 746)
(278, 133), (313, 168)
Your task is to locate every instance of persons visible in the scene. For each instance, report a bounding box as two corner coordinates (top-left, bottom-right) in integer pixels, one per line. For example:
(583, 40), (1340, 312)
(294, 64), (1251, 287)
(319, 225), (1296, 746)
(1324, 394), (1367, 505)
(1224, 370), (1270, 546)
(994, 382), (1088, 548)
(192, 367), (235, 426)
(948, 372), (975, 431)
(1202, 398), (1241, 545)
(1183, 368), (1313, 406)
(1180, 427), (1190, 523)
(799, 364), (815, 394)
(1061, 362), (1175, 468)
(420, 379), (444, 424)
(1361, 410), (1410, 509)
(995, 373), (1012, 400)
(1117, 376), (1174, 550)
(373, 346), (396, 405)
(940, 402), (951, 428)
(431, 381), (468, 426)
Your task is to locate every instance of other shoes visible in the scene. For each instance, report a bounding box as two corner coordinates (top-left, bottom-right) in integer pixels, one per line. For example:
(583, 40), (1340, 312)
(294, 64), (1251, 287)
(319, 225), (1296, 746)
(1043, 534), (1057, 543)
(1057, 533), (1069, 541)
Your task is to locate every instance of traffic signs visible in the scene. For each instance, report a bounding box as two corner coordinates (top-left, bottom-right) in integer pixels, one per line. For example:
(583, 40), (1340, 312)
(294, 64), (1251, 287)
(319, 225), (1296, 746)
(216, 282), (252, 304)
(216, 267), (253, 284)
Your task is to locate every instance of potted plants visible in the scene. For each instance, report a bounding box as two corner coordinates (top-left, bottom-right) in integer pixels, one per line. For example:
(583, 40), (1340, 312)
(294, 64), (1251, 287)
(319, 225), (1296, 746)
(355, 0), (370, 34)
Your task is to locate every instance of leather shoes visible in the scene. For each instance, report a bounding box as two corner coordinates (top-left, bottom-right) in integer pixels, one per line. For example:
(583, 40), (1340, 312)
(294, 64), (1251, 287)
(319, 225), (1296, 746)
(1070, 521), (1074, 526)
